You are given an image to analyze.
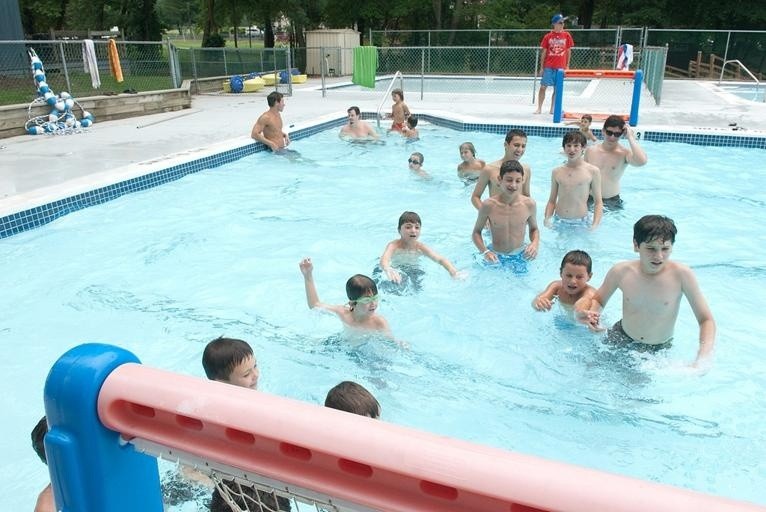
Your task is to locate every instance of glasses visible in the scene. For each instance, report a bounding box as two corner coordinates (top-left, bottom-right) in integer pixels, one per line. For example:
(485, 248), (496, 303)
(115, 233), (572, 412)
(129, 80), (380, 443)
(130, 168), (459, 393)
(352, 295), (383, 304)
(605, 128), (623, 137)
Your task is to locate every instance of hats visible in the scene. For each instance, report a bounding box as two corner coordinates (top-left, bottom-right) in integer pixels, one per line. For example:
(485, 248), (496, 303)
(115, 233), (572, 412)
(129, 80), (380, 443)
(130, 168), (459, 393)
(552, 14), (568, 25)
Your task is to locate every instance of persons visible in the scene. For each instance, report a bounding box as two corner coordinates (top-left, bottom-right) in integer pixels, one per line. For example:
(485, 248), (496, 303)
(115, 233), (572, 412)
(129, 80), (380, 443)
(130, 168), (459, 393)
(30, 416), (56, 512)
(407, 152), (427, 177)
(387, 88), (412, 133)
(210, 479), (292, 511)
(251, 91), (291, 154)
(159, 338), (260, 508)
(456, 114), (648, 278)
(338, 106), (380, 140)
(324, 380), (381, 420)
(529, 250), (600, 329)
(402, 115), (419, 142)
(532, 12), (574, 114)
(372, 210), (456, 297)
(299, 256), (410, 361)
(587, 214), (717, 375)
